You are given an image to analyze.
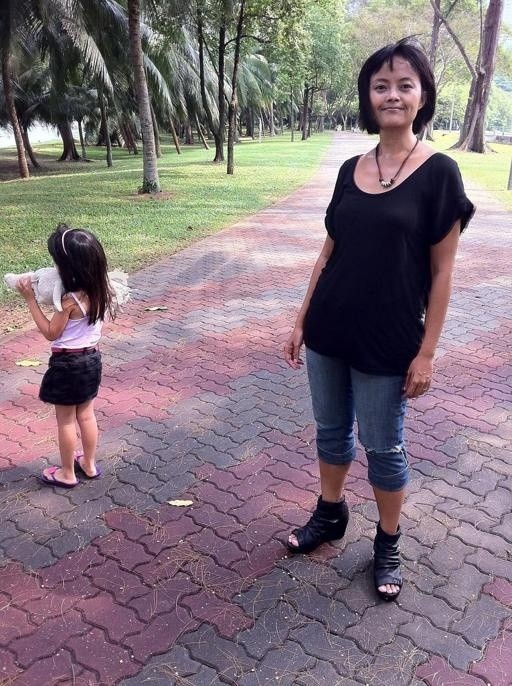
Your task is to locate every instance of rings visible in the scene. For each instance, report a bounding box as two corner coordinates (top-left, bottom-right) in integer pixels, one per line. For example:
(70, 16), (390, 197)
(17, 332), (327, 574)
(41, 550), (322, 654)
(423, 388), (428, 391)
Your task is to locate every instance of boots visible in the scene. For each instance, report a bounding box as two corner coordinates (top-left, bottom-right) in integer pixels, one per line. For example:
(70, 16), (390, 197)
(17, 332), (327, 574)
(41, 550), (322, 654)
(287, 494), (349, 554)
(373, 520), (403, 601)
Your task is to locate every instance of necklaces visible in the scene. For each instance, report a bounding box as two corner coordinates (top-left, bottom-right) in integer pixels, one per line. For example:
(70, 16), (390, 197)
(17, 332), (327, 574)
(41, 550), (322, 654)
(376, 137), (419, 187)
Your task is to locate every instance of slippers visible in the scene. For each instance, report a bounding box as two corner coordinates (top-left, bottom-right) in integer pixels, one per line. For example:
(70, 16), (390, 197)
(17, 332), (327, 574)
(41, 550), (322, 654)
(41, 467), (79, 487)
(74, 453), (101, 479)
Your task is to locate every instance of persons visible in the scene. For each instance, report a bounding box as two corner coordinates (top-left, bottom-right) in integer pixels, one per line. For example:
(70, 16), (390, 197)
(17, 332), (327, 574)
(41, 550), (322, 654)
(282, 31), (477, 597)
(16, 224), (118, 488)
